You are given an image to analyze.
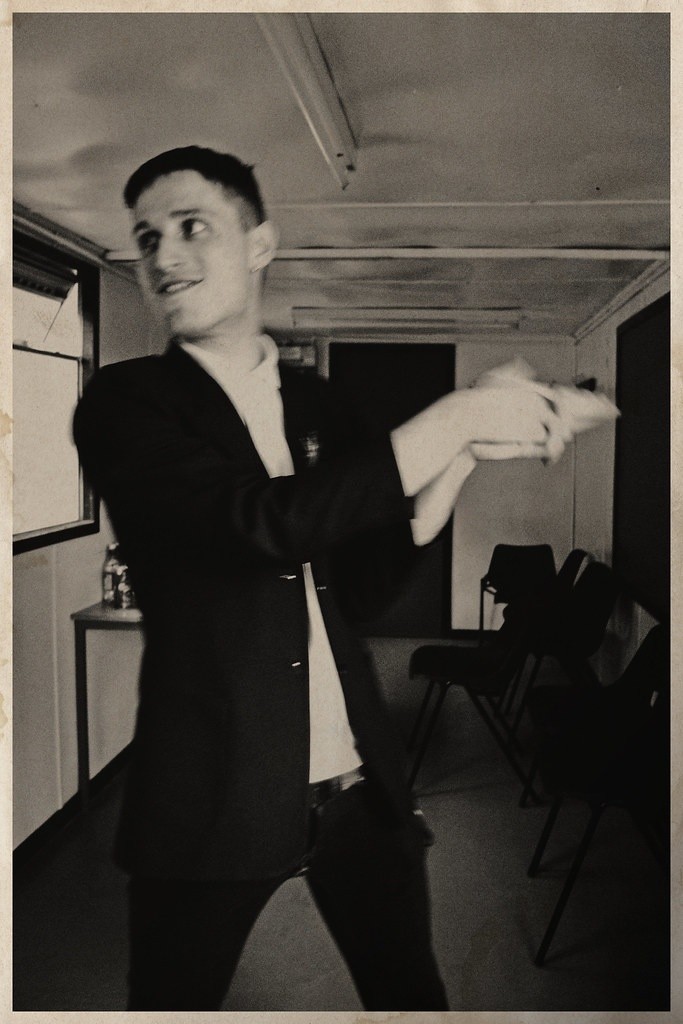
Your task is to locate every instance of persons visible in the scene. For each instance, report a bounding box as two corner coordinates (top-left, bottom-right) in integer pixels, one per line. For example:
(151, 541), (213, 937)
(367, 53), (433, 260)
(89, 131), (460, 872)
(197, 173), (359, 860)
(70, 145), (621, 1012)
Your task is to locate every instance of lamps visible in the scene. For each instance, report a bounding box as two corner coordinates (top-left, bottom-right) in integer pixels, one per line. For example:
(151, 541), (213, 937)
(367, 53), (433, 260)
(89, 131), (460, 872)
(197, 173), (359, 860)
(255, 14), (357, 193)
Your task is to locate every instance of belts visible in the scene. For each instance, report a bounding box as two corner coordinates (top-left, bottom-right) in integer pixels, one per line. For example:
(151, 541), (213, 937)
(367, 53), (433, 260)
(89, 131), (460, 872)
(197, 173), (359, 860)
(309, 763), (375, 810)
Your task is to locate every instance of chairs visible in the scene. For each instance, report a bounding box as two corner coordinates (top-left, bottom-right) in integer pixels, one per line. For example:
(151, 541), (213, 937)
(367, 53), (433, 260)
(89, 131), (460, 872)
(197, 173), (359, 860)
(407, 542), (669, 965)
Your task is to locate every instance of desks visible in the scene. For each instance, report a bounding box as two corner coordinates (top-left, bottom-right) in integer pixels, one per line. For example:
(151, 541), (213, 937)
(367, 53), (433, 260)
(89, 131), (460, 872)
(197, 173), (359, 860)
(69, 600), (146, 805)
